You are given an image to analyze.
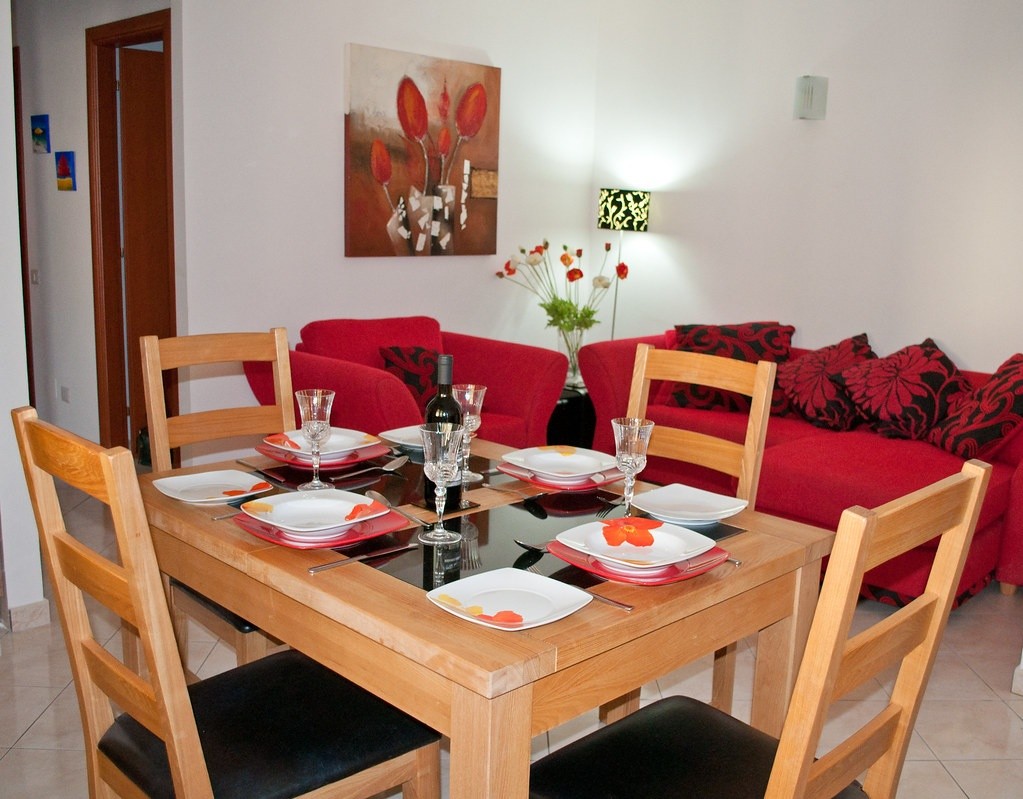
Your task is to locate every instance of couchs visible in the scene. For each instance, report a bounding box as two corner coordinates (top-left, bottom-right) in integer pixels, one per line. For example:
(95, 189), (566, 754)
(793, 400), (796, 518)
(576, 338), (1023, 635)
(240, 315), (568, 449)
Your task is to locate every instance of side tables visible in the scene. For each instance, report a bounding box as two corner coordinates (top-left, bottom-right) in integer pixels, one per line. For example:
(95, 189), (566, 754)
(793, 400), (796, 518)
(547, 387), (597, 449)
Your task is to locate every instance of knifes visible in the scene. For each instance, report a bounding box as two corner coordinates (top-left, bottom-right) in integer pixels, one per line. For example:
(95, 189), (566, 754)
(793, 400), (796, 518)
(308, 543), (419, 573)
(480, 468), (499, 474)
(237, 459), (286, 482)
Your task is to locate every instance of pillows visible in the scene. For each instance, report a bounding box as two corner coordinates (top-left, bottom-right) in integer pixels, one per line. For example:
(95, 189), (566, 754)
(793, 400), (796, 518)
(381, 346), (446, 410)
(655, 321), (780, 404)
(666, 324), (796, 419)
(925, 352), (1023, 460)
(829, 337), (974, 440)
(777, 332), (878, 431)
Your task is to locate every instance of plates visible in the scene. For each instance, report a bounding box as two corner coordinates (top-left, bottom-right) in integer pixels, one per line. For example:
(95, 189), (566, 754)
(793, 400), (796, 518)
(497, 445), (625, 492)
(152, 470), (273, 505)
(379, 424), (477, 451)
(232, 489), (410, 549)
(631, 483), (749, 525)
(547, 518), (731, 586)
(426, 567), (594, 631)
(256, 427), (391, 471)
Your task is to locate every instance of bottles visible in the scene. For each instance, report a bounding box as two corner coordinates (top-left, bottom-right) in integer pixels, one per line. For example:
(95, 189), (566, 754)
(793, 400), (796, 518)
(423, 516), (461, 592)
(424, 356), (463, 506)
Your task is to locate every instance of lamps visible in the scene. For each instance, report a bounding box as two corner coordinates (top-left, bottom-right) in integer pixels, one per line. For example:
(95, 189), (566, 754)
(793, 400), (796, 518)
(596, 188), (650, 340)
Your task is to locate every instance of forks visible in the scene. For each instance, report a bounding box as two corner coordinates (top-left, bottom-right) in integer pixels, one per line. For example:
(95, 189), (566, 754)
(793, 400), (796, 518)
(597, 495), (625, 504)
(524, 562), (635, 611)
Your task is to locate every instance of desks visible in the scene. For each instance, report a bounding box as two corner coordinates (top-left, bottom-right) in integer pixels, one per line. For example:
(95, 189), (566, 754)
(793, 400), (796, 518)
(115, 429), (844, 799)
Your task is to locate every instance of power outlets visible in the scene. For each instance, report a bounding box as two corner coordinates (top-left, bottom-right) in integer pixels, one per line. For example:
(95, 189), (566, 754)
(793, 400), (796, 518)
(60, 386), (72, 403)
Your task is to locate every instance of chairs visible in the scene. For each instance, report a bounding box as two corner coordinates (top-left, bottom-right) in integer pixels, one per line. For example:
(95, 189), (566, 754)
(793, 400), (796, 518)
(568, 344), (775, 722)
(12, 405), (443, 798)
(531, 461), (991, 798)
(124, 323), (307, 683)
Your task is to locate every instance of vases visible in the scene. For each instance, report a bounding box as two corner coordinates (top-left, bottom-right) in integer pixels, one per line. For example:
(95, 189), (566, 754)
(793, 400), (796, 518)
(563, 325), (587, 386)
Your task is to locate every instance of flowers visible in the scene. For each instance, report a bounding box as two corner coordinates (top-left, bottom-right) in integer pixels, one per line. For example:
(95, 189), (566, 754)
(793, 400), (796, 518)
(495, 243), (629, 330)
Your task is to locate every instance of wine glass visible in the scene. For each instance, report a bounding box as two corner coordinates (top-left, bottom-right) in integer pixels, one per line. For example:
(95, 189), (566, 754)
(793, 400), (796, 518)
(418, 424), (465, 544)
(611, 418), (655, 517)
(461, 515), (483, 570)
(452, 384), (487, 482)
(295, 389), (335, 491)
(433, 545), (445, 589)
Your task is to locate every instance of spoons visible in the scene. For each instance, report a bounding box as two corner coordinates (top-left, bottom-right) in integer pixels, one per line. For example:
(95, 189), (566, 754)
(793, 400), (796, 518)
(365, 487), (434, 530)
(330, 456), (409, 480)
(482, 483), (548, 500)
(514, 539), (556, 551)
